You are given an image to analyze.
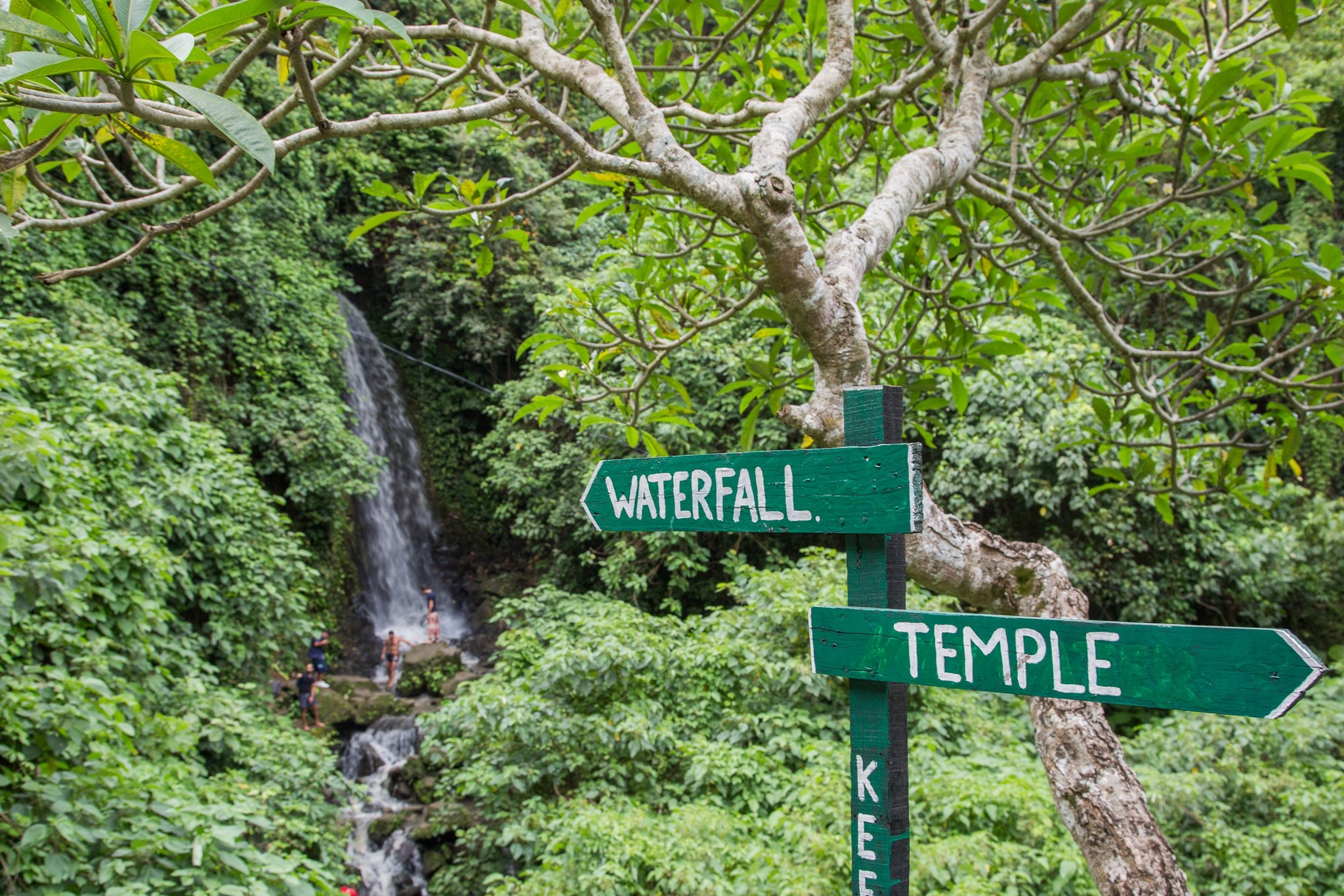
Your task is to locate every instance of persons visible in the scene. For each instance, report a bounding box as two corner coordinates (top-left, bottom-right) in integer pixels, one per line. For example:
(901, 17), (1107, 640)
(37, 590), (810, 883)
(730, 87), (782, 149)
(292, 662), (326, 732)
(307, 630), (333, 688)
(379, 630), (416, 690)
(419, 583), (441, 644)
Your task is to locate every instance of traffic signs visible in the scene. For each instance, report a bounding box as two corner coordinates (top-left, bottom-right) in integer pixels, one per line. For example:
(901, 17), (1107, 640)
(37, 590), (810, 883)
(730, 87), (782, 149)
(809, 605), (1330, 720)
(579, 437), (925, 535)
